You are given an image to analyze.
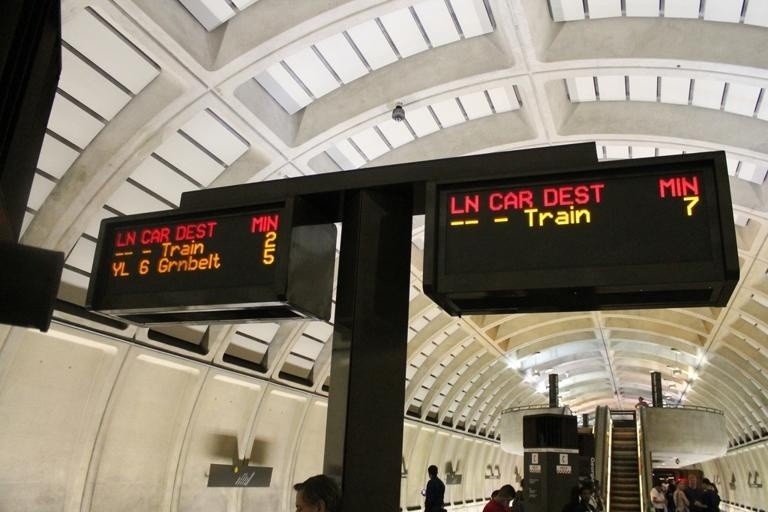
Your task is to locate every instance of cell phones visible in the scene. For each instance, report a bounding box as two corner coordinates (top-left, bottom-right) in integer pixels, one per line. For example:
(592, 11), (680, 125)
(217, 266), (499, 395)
(422, 489), (426, 496)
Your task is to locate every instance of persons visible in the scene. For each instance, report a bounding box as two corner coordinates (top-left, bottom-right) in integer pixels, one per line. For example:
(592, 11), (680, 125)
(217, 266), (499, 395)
(636, 396), (649, 408)
(424, 465), (447, 511)
(483, 479), (606, 512)
(294, 475), (340, 512)
(650, 475), (722, 511)
